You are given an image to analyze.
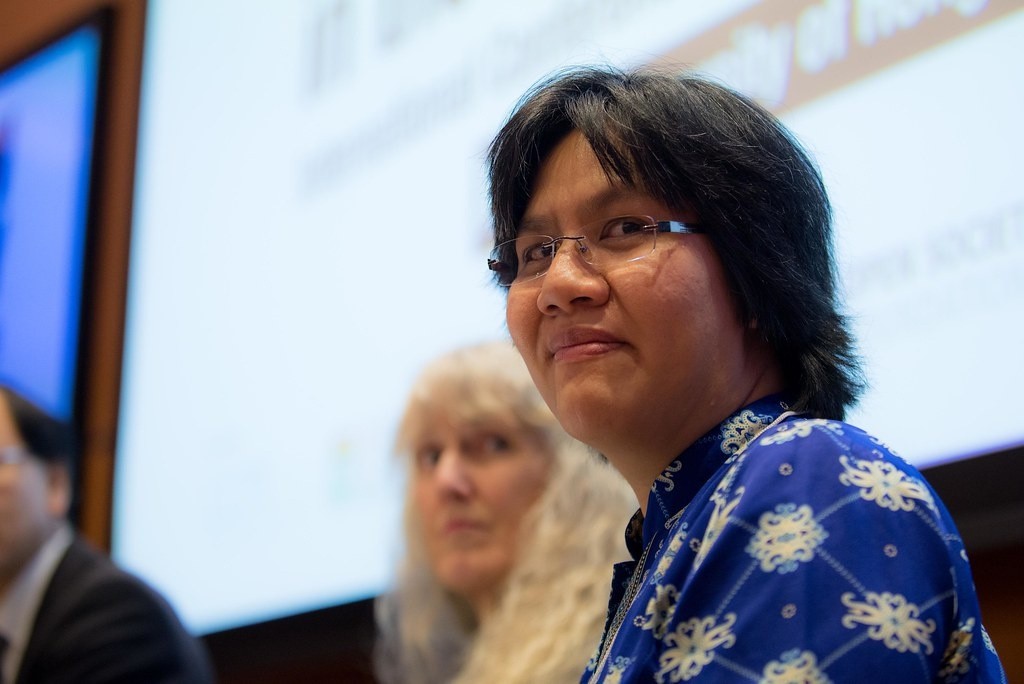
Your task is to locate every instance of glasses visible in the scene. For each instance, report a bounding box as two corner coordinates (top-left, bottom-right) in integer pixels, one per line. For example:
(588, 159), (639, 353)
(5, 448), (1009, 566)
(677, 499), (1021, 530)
(487, 212), (699, 286)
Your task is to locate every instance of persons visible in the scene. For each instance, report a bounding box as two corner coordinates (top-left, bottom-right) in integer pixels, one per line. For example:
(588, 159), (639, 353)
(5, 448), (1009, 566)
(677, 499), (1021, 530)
(474, 56), (1008, 684)
(0, 382), (220, 684)
(370, 335), (644, 684)
